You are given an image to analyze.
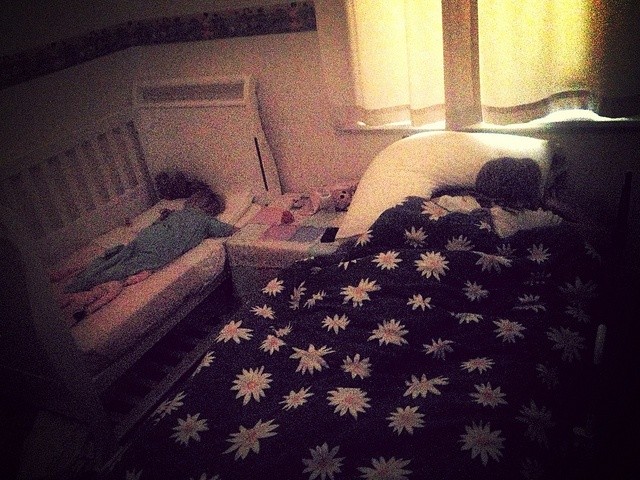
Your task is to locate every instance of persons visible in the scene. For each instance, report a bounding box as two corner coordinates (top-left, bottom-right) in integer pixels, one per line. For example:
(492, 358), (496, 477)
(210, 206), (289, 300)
(474, 158), (532, 210)
(47, 189), (239, 318)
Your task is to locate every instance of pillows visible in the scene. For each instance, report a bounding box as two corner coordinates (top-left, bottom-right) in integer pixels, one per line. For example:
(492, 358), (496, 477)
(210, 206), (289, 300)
(333, 132), (555, 244)
(214, 190), (259, 224)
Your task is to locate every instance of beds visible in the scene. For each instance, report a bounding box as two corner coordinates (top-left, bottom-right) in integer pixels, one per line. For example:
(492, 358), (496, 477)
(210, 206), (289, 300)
(1, 186), (262, 426)
(101, 122), (639, 479)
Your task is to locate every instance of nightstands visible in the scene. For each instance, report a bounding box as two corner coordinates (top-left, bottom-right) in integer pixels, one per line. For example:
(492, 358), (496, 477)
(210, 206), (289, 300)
(224, 192), (349, 308)
